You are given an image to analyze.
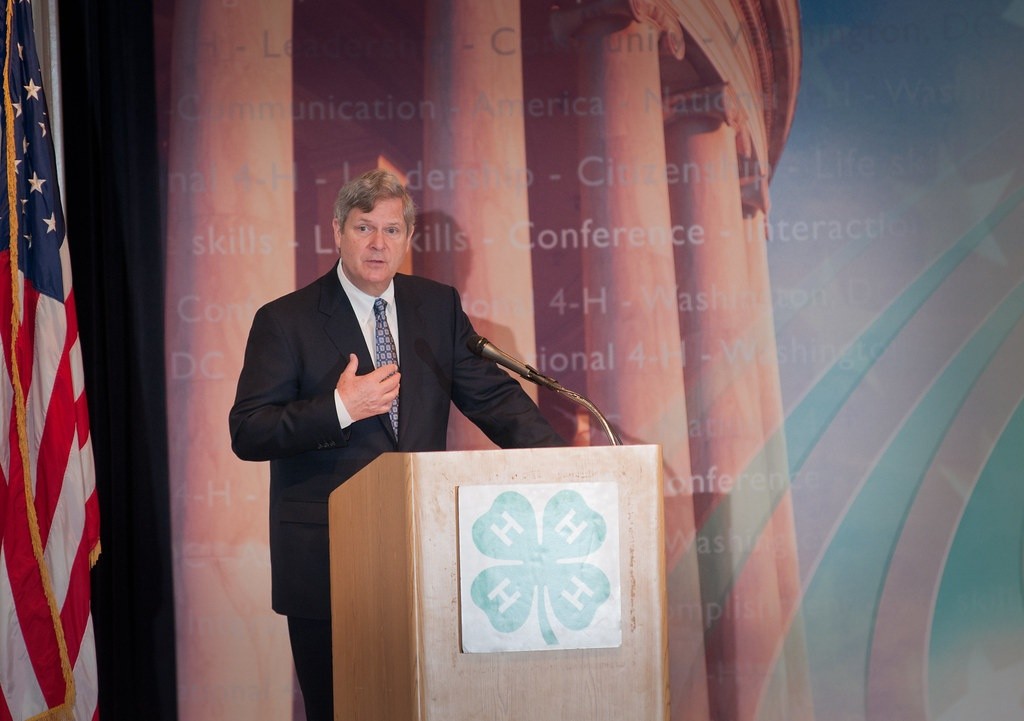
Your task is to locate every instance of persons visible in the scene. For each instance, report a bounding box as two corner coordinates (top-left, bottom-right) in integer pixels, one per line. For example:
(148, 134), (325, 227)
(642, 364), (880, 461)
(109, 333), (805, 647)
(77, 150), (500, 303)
(228, 170), (567, 721)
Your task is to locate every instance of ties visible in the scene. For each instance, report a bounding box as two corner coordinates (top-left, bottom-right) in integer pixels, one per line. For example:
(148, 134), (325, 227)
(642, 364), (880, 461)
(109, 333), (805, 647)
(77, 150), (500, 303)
(372, 298), (399, 442)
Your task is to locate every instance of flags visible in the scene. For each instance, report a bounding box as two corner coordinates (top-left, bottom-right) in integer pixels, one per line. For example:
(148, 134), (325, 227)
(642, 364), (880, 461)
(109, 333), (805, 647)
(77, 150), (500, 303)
(0, 0), (102, 721)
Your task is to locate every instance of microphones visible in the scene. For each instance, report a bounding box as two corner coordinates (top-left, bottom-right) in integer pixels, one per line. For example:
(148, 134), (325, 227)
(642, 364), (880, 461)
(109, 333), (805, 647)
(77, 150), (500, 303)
(466, 335), (564, 393)
